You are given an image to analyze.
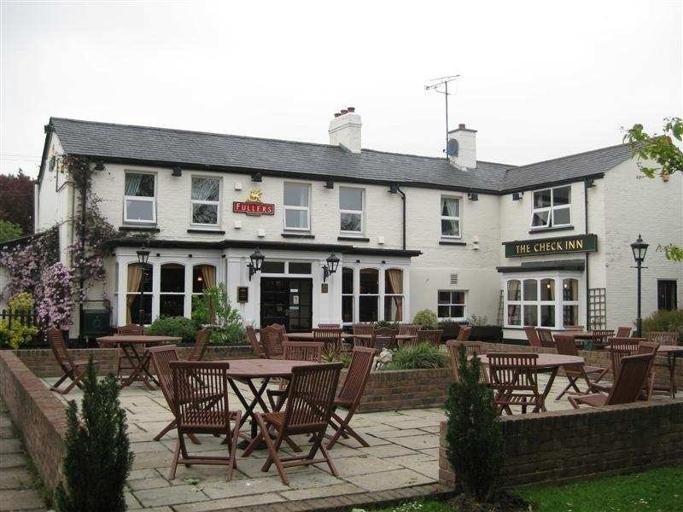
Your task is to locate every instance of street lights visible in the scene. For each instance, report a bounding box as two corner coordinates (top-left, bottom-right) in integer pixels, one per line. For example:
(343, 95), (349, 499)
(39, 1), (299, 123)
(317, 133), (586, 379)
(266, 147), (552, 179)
(629, 235), (649, 334)
(135, 245), (149, 330)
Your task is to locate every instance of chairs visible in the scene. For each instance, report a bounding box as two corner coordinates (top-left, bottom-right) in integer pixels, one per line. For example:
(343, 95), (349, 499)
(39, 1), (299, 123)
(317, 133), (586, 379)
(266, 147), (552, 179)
(49, 318), (212, 392)
(446, 326), (682, 414)
(266, 341), (326, 411)
(165, 360), (243, 482)
(240, 361), (348, 486)
(307, 346), (377, 450)
(245, 320), (472, 359)
(147, 344), (223, 446)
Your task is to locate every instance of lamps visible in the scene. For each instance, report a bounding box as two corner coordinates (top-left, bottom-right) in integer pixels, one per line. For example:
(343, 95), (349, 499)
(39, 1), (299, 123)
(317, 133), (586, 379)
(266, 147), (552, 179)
(245, 247), (264, 282)
(321, 250), (340, 284)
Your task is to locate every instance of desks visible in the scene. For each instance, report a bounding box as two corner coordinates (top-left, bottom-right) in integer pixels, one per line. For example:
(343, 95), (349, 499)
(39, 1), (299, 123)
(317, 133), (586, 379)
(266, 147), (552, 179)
(192, 357), (320, 459)
